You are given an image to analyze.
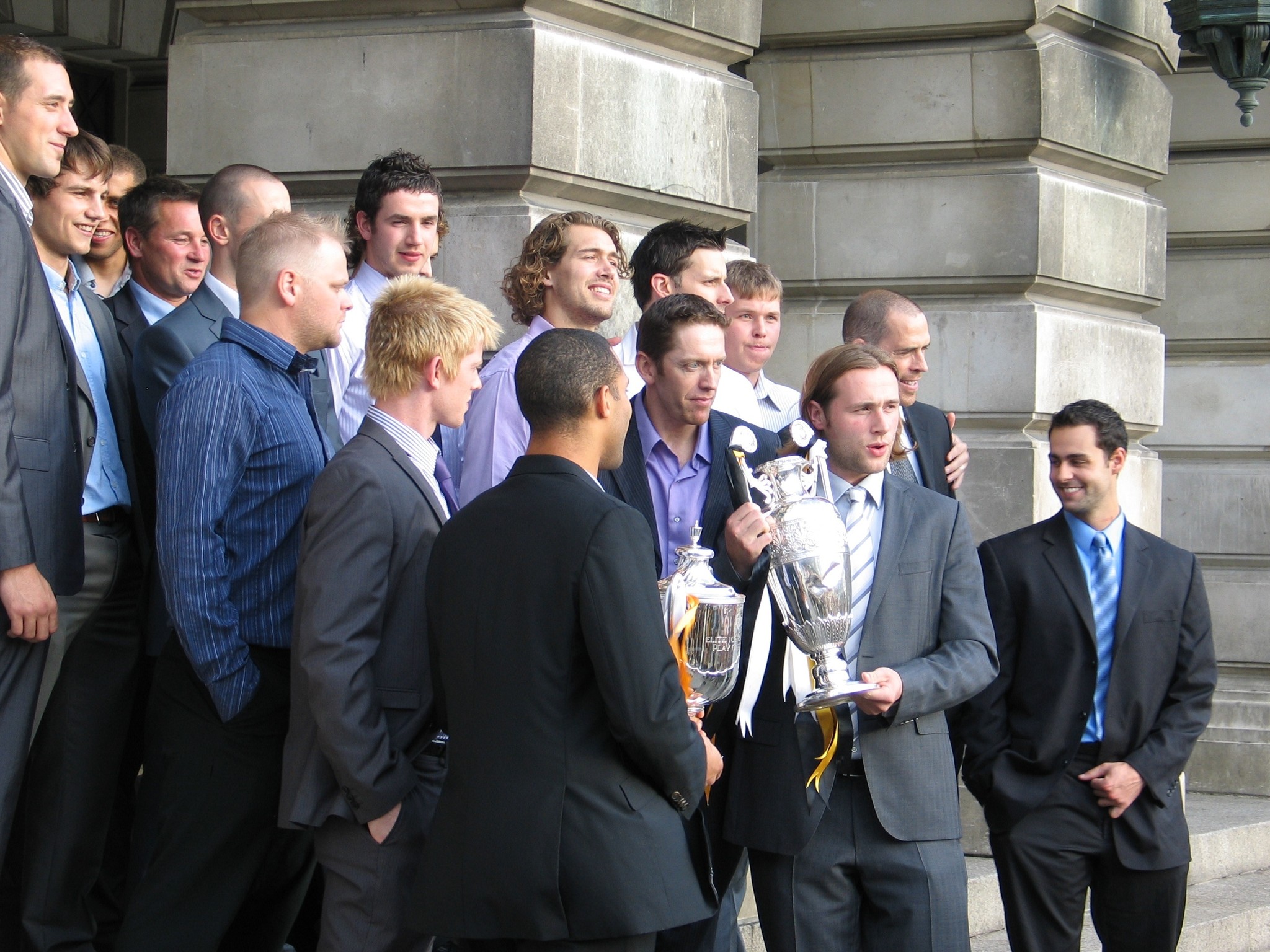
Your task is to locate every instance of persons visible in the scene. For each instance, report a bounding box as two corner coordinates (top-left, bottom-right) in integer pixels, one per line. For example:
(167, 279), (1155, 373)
(0, 35), (292, 952)
(275, 272), (502, 952)
(708, 341), (1000, 952)
(322, 152), (466, 490)
(948, 401), (1216, 952)
(599, 219), (969, 952)
(457, 211), (637, 510)
(127, 210), (354, 952)
(402, 327), (724, 952)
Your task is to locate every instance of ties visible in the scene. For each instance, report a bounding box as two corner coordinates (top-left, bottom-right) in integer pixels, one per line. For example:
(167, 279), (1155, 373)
(435, 453), (461, 517)
(844, 486), (876, 742)
(890, 440), (920, 485)
(1090, 532), (1120, 740)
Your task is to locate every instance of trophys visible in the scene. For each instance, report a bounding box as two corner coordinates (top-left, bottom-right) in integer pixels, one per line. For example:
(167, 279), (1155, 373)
(658, 517), (747, 717)
(727, 419), (880, 711)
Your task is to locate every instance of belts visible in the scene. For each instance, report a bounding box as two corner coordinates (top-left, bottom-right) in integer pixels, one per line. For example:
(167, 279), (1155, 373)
(836, 771), (866, 783)
(83, 505), (136, 527)
